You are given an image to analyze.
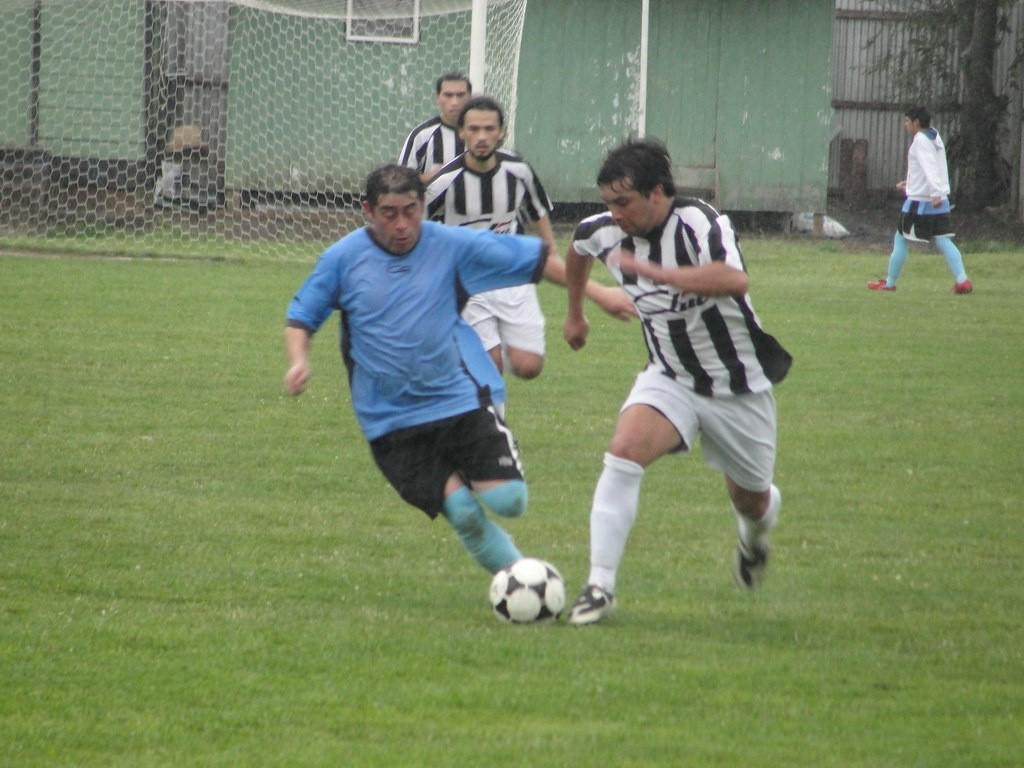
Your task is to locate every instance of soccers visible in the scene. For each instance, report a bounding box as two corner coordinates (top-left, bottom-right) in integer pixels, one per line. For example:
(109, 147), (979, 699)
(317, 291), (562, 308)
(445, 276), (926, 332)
(491, 558), (565, 623)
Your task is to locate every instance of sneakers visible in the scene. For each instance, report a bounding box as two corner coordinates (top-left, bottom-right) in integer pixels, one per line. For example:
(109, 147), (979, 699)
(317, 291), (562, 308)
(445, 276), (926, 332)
(868, 279), (896, 292)
(955, 280), (974, 293)
(565, 586), (619, 626)
(736, 541), (769, 588)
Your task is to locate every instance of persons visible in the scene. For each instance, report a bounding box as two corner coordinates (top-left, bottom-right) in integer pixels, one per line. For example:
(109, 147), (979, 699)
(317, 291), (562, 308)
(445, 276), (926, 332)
(864, 106), (972, 294)
(567, 137), (790, 626)
(396, 73), (471, 180)
(424, 95), (556, 420)
(285, 166), (639, 578)
